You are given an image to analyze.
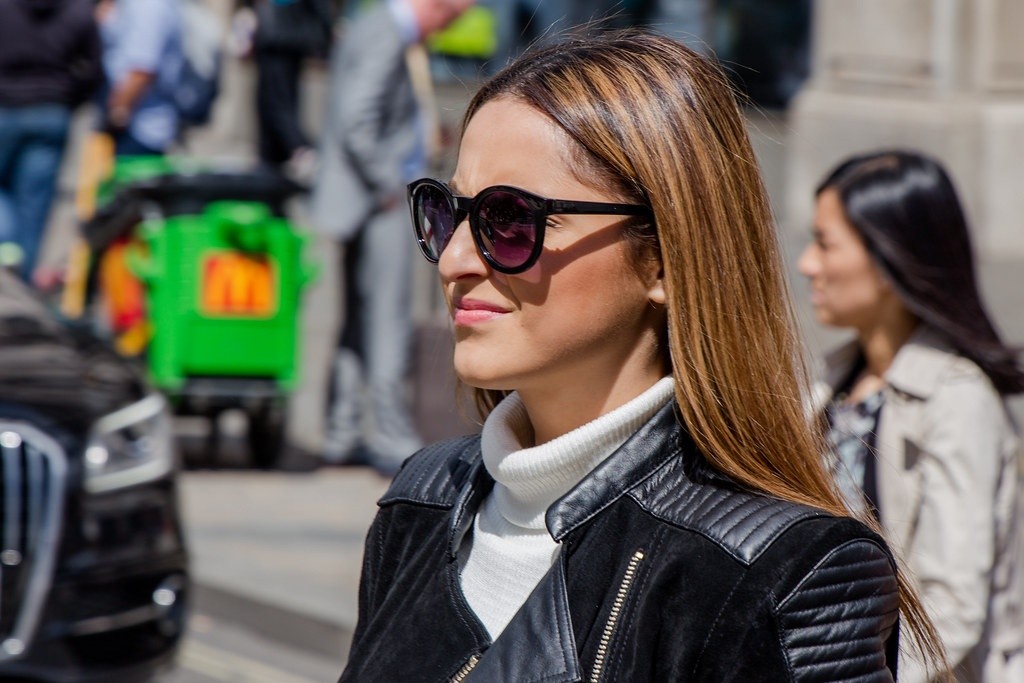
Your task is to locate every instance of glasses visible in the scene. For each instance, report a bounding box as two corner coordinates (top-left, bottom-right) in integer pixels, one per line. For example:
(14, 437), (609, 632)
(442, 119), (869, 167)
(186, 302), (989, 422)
(406, 177), (656, 274)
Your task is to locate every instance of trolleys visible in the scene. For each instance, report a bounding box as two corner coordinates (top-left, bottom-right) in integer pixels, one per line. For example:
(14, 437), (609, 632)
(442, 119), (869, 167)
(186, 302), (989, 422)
(99, 157), (303, 467)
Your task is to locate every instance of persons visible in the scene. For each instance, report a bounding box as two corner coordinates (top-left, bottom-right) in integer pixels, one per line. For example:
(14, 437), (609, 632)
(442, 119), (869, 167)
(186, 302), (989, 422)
(337, 24), (955, 683)
(796, 146), (1024, 683)
(0, 0), (824, 475)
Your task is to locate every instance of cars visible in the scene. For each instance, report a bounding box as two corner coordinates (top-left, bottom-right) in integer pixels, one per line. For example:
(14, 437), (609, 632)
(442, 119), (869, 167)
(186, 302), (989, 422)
(0, 268), (189, 683)
